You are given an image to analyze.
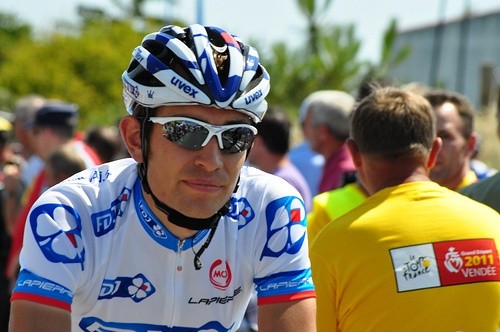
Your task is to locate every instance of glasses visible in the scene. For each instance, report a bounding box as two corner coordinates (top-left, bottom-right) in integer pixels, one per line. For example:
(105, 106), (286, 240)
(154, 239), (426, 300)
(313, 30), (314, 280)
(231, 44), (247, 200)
(136, 116), (259, 154)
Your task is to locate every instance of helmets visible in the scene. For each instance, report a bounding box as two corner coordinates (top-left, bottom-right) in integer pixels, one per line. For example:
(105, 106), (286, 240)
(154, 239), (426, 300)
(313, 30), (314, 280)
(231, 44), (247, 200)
(121, 24), (270, 124)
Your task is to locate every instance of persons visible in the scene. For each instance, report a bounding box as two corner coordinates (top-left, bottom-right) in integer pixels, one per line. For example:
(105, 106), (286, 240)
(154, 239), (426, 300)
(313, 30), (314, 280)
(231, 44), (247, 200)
(0, 83), (500, 332)
(7, 23), (317, 332)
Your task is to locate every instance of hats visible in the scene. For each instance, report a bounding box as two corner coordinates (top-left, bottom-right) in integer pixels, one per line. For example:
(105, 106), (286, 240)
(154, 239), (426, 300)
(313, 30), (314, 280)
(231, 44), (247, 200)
(34, 104), (76, 126)
